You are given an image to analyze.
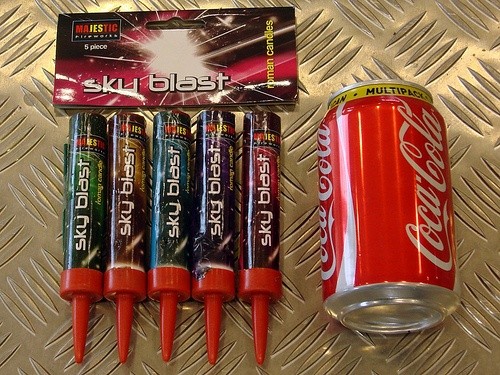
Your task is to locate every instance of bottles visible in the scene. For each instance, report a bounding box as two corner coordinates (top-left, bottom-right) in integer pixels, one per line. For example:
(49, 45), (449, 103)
(148, 109), (190, 361)
(238, 109), (282, 365)
(59, 111), (106, 365)
(104, 113), (148, 364)
(191, 109), (237, 364)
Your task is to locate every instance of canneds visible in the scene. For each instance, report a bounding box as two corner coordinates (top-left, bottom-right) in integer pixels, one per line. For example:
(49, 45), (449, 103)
(317, 78), (461, 334)
